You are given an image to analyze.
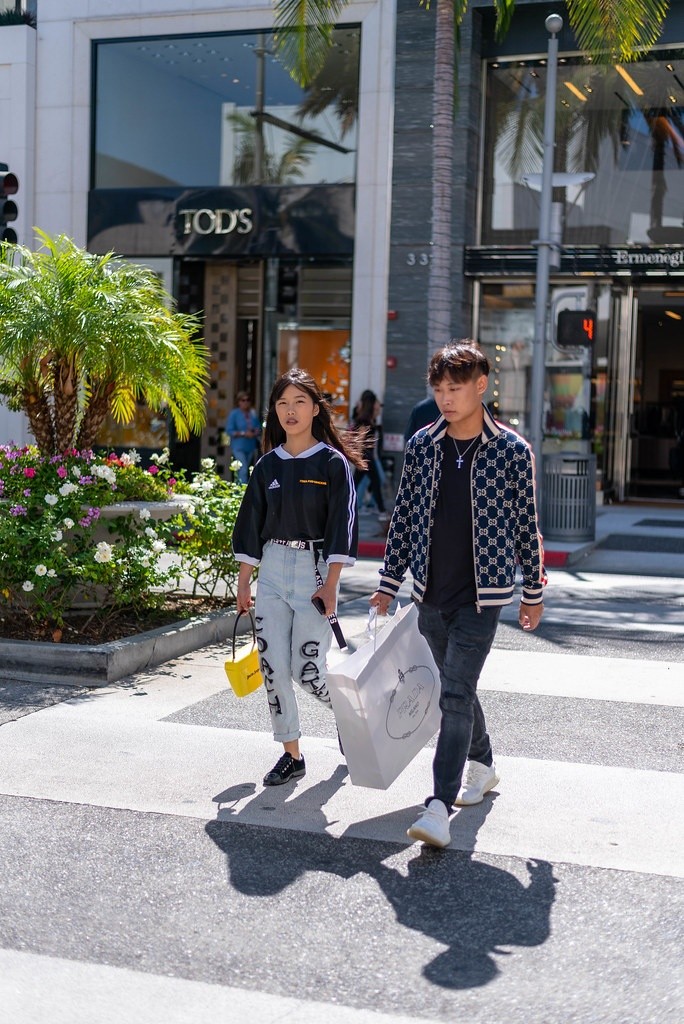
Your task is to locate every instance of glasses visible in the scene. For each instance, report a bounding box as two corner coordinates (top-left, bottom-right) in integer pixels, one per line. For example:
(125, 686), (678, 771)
(240, 398), (250, 402)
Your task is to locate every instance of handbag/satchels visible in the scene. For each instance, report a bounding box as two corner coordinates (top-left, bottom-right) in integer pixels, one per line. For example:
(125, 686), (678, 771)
(324, 603), (444, 789)
(223, 610), (265, 697)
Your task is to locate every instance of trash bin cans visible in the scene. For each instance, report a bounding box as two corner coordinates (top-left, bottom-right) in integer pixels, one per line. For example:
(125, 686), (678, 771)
(381, 415), (413, 513)
(541, 452), (597, 543)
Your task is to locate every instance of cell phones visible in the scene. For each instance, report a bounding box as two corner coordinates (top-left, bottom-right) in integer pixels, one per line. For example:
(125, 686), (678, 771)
(311, 596), (326, 615)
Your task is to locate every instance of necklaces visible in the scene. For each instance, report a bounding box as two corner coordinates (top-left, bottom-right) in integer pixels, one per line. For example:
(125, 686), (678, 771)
(448, 425), (482, 469)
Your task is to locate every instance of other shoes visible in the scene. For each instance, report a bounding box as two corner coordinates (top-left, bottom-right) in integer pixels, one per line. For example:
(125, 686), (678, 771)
(358, 508), (372, 515)
(378, 512), (392, 521)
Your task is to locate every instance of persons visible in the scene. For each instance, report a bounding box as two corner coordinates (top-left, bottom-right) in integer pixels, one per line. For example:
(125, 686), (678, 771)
(368, 340), (546, 850)
(232, 368), (359, 786)
(403, 395), (442, 447)
(227, 389), (264, 487)
(346, 389), (392, 521)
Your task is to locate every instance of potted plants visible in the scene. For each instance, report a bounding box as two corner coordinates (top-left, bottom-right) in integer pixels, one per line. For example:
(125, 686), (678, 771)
(0, 223), (205, 603)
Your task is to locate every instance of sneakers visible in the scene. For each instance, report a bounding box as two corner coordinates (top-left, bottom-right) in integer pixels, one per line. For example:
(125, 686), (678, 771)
(454, 759), (500, 805)
(407, 798), (451, 847)
(336, 725), (344, 756)
(263, 751), (305, 785)
